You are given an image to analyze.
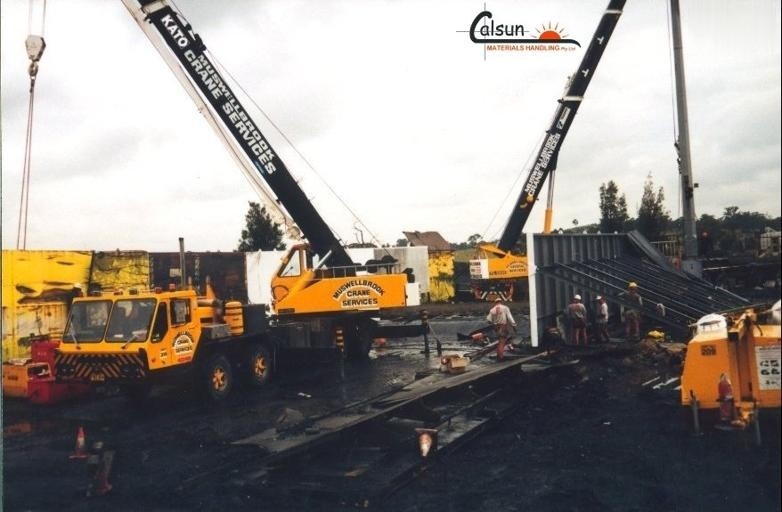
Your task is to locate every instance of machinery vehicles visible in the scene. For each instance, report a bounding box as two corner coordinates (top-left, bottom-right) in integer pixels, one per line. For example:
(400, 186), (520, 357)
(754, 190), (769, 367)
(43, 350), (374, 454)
(52, 244), (409, 406)
(466, 241), (528, 302)
(675, 291), (781, 434)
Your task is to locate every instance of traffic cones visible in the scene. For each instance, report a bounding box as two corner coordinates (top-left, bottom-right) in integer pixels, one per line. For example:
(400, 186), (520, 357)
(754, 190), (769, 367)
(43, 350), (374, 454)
(68, 425), (92, 461)
(412, 427), (441, 462)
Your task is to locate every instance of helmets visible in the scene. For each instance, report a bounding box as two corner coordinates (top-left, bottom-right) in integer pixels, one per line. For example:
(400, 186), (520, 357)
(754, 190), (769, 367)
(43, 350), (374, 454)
(574, 294), (582, 300)
(595, 295), (602, 300)
(628, 281), (639, 288)
(495, 297), (502, 303)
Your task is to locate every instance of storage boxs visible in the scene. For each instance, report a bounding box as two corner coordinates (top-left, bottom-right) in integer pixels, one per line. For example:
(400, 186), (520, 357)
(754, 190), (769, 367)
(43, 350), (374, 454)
(448, 357), (465, 374)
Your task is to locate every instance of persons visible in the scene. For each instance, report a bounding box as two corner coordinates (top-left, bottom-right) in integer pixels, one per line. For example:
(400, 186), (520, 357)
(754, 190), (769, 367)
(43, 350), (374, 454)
(718, 372), (733, 421)
(567, 294), (587, 345)
(487, 298), (518, 361)
(618, 282), (643, 341)
(595, 295), (610, 342)
